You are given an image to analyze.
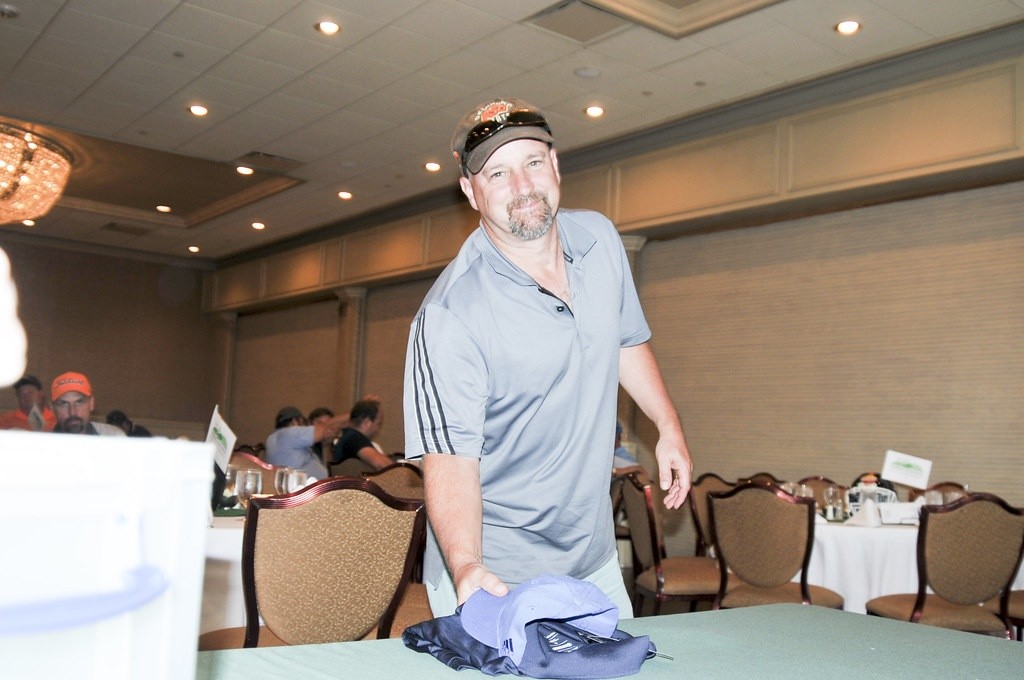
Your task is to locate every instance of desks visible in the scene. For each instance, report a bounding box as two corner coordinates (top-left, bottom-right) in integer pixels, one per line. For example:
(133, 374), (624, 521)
(783, 502), (1024, 620)
(203, 514), (261, 635)
(193, 602), (1023, 680)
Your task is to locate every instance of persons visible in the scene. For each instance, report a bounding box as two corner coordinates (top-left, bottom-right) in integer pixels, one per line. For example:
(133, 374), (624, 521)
(0, 374), (58, 431)
(306, 407), (340, 461)
(332, 400), (397, 472)
(403, 96), (693, 619)
(49, 371), (126, 436)
(106, 409), (152, 437)
(265, 395), (379, 480)
(614, 418), (638, 464)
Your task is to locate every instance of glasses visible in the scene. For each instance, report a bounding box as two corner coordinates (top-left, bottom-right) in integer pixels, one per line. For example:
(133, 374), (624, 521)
(462, 108), (553, 179)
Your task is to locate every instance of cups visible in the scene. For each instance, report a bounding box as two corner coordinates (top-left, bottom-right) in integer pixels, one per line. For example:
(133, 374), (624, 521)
(776, 483), (816, 498)
(275, 470), (307, 494)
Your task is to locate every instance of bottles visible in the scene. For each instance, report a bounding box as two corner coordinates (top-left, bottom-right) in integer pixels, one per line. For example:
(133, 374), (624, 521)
(860, 476), (878, 507)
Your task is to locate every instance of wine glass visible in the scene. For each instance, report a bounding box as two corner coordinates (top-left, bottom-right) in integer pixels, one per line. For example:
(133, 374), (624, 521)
(233, 468), (263, 522)
(220, 464), (238, 511)
(824, 484), (840, 520)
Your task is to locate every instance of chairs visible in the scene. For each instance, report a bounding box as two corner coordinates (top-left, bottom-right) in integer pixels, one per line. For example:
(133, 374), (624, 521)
(222, 443), (431, 648)
(608, 467), (1024, 641)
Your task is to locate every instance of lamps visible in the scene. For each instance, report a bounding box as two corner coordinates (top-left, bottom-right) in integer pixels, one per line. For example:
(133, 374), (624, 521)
(0, 121), (75, 226)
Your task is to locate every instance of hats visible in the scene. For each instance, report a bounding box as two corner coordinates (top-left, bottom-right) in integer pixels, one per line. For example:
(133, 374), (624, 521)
(106, 411), (126, 425)
(274, 407), (307, 427)
(14, 376), (41, 390)
(462, 573), (617, 666)
(451, 98), (553, 174)
(50, 371), (91, 404)
